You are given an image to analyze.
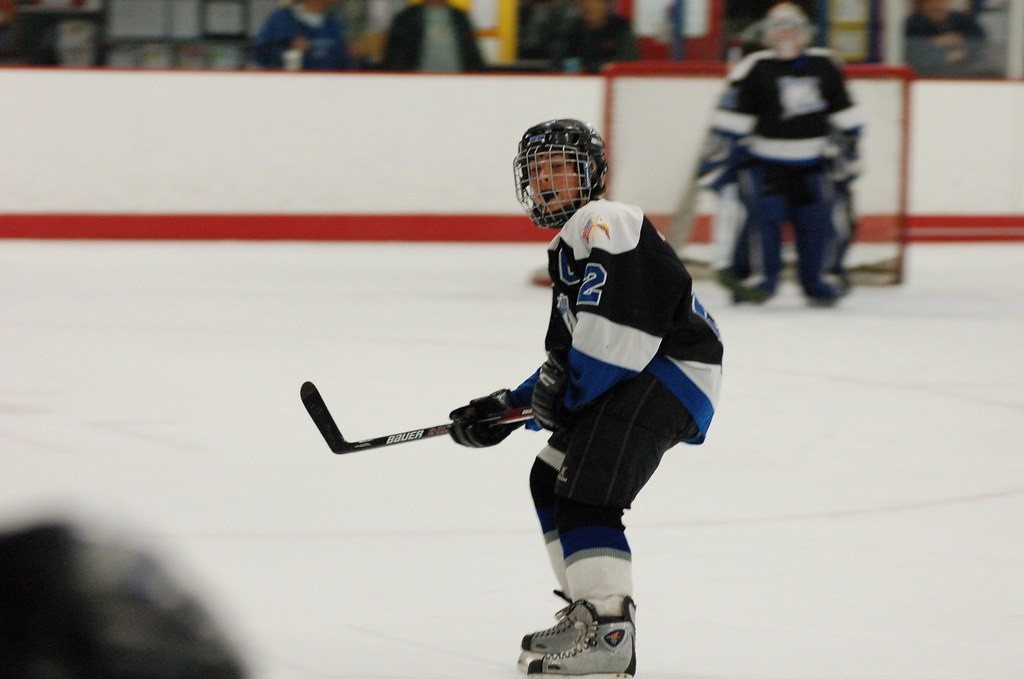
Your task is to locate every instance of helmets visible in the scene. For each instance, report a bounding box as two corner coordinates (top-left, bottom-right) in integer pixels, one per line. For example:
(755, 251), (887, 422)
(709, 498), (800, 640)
(521, 120), (609, 201)
(760, 5), (814, 44)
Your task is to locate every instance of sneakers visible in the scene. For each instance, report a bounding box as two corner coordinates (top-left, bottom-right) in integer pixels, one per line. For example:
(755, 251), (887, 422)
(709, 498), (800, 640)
(521, 590), (587, 654)
(517, 595), (636, 679)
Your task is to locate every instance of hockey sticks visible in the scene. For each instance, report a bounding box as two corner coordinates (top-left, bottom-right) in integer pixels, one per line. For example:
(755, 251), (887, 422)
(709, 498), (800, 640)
(708, 34), (773, 305)
(301, 381), (537, 456)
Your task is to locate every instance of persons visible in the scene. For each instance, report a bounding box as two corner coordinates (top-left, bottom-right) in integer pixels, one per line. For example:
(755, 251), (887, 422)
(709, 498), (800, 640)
(449, 120), (724, 679)
(695, 4), (863, 309)
(0, 0), (1003, 76)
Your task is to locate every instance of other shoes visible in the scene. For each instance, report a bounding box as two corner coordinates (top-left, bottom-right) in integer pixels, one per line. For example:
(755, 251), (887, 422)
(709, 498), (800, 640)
(807, 273), (843, 301)
(731, 274), (777, 302)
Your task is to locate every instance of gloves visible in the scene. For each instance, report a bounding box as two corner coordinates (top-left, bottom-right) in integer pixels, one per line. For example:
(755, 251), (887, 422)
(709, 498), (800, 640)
(448, 389), (526, 449)
(696, 134), (734, 192)
(826, 132), (862, 183)
(531, 363), (569, 432)
